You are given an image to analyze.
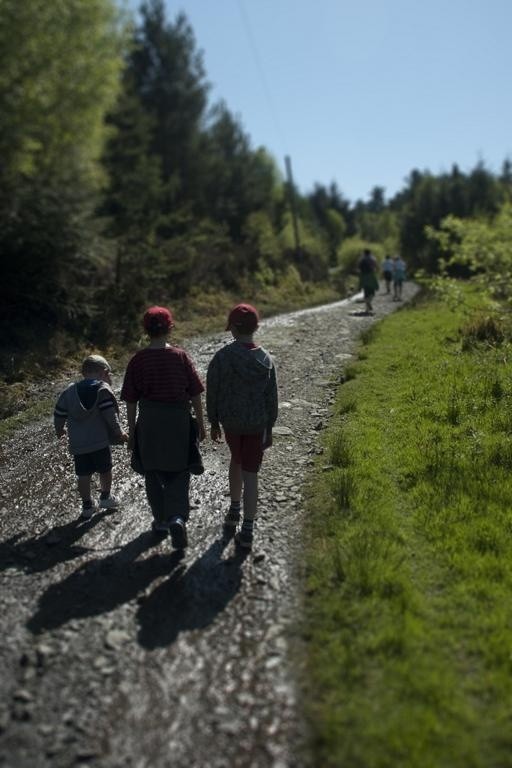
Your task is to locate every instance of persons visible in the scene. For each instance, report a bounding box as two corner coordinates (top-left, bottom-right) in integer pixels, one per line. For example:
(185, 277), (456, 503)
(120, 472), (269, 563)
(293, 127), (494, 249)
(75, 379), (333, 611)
(119, 305), (205, 547)
(391, 253), (408, 300)
(360, 248), (378, 309)
(209, 303), (277, 549)
(54, 355), (129, 518)
(383, 255), (394, 294)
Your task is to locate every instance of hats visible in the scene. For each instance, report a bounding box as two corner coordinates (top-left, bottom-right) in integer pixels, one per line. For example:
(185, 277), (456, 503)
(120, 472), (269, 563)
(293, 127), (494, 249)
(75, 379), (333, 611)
(225, 304), (257, 330)
(143, 307), (171, 327)
(85, 355), (111, 385)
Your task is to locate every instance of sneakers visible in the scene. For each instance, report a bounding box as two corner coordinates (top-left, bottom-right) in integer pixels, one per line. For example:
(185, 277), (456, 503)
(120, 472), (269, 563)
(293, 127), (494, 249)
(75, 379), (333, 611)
(225, 511), (240, 525)
(152, 520), (167, 532)
(99, 495), (120, 507)
(170, 516), (186, 548)
(82, 499), (96, 517)
(236, 532), (252, 547)
